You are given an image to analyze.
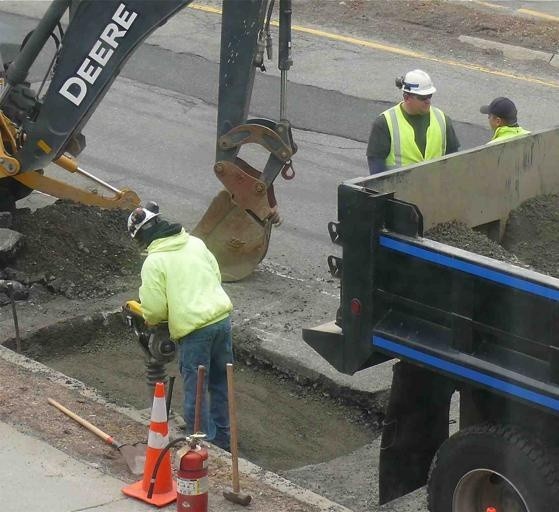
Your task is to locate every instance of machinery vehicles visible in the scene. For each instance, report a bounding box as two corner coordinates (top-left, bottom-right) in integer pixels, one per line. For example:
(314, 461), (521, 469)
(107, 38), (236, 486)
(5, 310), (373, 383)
(0, 0), (298, 283)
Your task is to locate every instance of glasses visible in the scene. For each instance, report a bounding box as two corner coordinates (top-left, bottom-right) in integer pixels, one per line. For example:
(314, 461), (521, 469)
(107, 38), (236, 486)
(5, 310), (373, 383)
(407, 94), (432, 102)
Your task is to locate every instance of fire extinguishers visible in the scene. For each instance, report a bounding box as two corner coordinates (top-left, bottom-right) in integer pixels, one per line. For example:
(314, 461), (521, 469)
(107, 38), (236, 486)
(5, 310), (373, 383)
(146, 432), (209, 512)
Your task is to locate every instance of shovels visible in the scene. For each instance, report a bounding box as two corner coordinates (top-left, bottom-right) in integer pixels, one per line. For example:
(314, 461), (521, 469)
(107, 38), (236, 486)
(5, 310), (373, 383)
(47, 397), (146, 475)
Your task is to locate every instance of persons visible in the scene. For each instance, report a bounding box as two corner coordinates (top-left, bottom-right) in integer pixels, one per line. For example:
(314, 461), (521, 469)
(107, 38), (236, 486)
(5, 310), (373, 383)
(127, 201), (234, 452)
(478, 95), (530, 145)
(367, 68), (460, 176)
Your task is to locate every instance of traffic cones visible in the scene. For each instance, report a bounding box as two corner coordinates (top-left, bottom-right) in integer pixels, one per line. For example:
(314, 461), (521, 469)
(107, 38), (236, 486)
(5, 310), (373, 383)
(121, 382), (177, 508)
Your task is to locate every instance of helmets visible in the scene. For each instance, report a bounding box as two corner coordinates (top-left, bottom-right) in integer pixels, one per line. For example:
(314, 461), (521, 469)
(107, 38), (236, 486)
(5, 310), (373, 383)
(127, 201), (163, 240)
(395, 70), (436, 96)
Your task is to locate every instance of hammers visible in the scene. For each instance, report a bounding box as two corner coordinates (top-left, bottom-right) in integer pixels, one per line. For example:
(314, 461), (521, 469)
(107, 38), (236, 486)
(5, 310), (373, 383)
(223, 363), (252, 506)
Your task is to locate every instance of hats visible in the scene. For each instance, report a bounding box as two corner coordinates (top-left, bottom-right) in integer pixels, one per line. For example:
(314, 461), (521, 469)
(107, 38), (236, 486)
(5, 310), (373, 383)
(479, 97), (517, 125)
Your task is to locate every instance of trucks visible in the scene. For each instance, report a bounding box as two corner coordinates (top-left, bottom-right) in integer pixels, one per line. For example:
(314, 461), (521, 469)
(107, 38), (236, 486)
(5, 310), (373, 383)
(302, 126), (558, 511)
